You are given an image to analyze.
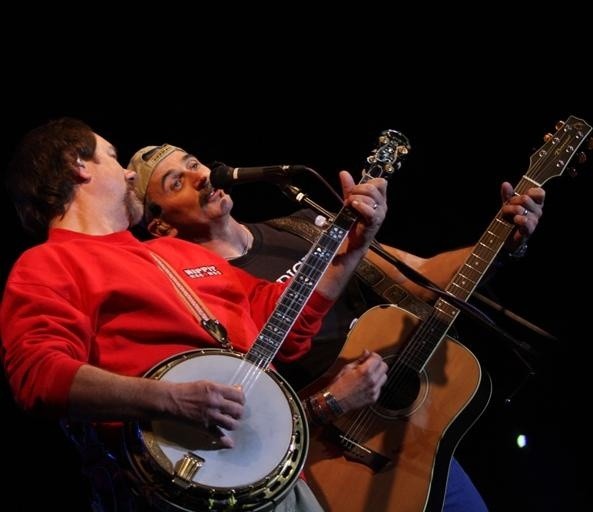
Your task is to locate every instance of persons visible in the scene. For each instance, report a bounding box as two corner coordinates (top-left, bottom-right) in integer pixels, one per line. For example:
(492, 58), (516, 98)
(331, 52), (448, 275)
(126, 137), (548, 512)
(0, 113), (389, 512)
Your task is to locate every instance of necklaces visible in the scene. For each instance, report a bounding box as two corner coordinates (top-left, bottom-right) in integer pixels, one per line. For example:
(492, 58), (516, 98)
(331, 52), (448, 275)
(224, 229), (249, 259)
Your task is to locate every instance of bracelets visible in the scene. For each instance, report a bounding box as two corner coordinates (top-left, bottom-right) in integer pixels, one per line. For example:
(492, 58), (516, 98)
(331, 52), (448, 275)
(299, 385), (344, 428)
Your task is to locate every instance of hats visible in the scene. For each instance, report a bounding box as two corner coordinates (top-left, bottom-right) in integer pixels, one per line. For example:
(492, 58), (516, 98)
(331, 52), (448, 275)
(126, 143), (185, 203)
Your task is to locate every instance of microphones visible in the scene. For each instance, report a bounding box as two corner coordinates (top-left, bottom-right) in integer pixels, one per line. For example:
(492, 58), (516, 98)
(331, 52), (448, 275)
(207, 164), (305, 188)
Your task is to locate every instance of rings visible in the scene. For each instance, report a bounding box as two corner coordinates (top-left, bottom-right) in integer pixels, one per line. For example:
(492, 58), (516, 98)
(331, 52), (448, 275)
(371, 202), (378, 210)
(521, 208), (528, 219)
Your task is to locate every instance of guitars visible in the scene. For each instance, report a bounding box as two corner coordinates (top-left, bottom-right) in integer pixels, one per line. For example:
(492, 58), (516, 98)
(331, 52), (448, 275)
(295, 115), (592, 512)
